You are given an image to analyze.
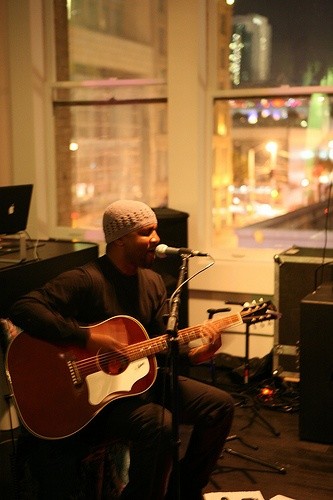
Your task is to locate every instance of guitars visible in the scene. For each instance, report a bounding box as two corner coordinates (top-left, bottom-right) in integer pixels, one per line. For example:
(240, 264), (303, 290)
(5, 297), (281, 442)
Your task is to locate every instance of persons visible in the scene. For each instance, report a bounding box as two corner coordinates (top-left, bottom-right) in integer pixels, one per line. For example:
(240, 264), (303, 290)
(8, 198), (235, 500)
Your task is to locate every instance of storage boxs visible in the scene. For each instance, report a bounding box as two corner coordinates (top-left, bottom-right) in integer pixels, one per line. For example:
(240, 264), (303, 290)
(273, 245), (333, 382)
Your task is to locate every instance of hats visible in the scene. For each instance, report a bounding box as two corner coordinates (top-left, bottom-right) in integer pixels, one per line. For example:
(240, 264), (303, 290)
(102, 199), (157, 243)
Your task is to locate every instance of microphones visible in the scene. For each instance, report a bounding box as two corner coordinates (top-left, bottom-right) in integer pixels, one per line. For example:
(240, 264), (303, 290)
(156, 244), (209, 259)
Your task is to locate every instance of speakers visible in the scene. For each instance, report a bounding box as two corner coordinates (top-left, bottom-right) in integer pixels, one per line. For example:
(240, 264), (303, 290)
(148, 207), (191, 379)
(298, 283), (333, 445)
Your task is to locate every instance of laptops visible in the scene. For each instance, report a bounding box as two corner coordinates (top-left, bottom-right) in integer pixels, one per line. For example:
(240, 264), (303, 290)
(0, 184), (33, 236)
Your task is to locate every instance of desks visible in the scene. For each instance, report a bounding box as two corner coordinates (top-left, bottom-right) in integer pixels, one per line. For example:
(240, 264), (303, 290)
(0, 238), (98, 318)
